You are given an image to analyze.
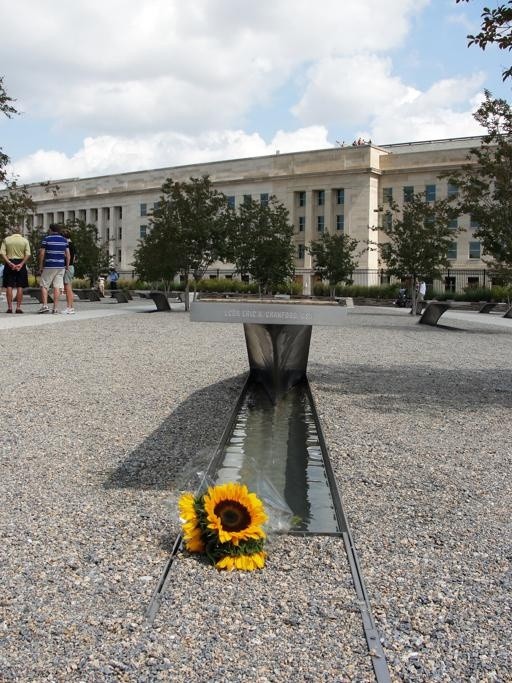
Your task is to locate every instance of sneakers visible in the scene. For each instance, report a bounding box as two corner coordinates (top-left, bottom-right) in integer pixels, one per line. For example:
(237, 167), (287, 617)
(16, 309), (23, 312)
(61, 307), (74, 314)
(52, 309), (58, 313)
(39, 304), (49, 312)
(7, 308), (12, 312)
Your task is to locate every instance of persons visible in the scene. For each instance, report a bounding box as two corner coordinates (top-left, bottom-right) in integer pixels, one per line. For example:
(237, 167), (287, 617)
(109, 268), (119, 298)
(37, 223), (71, 314)
(0, 224), (32, 313)
(0, 259), (5, 299)
(96, 272), (105, 297)
(60, 230), (76, 315)
(417, 279), (426, 300)
